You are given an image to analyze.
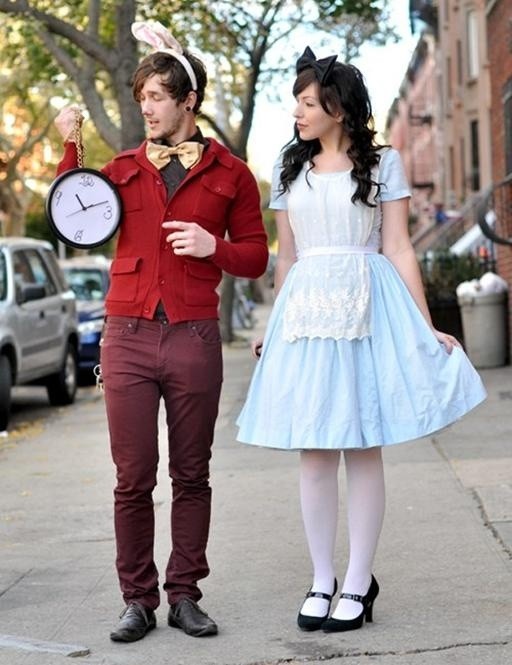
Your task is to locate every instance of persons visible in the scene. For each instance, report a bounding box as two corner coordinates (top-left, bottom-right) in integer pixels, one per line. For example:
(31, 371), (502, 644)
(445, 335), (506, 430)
(429, 203), (448, 225)
(56, 53), (269, 643)
(235, 62), (488, 633)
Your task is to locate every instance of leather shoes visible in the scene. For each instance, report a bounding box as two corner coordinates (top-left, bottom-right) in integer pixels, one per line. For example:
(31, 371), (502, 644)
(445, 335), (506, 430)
(110, 601), (157, 643)
(167, 599), (218, 637)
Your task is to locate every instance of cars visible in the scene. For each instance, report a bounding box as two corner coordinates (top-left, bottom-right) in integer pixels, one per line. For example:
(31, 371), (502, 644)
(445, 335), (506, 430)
(0, 235), (114, 431)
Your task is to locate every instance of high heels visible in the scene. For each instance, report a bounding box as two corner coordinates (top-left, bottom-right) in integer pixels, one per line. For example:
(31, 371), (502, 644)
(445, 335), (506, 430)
(322, 574), (379, 633)
(297, 577), (337, 631)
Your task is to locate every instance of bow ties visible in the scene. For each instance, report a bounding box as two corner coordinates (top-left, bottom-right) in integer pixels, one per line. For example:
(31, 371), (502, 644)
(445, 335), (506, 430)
(145, 140), (205, 170)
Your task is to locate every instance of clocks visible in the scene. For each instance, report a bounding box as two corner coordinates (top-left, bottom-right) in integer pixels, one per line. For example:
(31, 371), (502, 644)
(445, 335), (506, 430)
(43, 168), (122, 249)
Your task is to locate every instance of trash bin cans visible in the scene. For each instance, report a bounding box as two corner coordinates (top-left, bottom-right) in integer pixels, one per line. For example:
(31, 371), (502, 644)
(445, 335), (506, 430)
(456, 292), (506, 369)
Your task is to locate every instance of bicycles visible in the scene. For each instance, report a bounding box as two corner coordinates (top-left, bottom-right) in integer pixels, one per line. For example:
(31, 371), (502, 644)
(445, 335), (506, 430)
(235, 283), (260, 330)
